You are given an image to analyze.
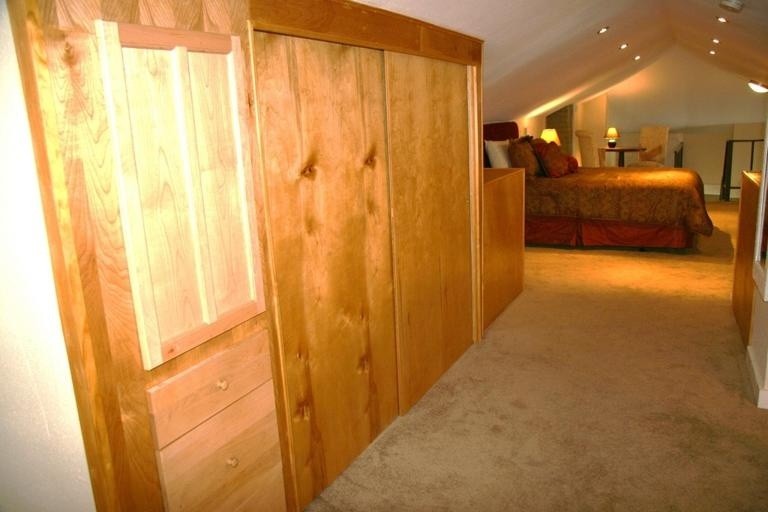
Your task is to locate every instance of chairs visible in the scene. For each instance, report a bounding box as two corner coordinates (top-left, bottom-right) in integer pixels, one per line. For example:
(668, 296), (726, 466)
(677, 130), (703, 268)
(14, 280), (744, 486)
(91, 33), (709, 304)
(576, 130), (600, 168)
(627, 126), (672, 166)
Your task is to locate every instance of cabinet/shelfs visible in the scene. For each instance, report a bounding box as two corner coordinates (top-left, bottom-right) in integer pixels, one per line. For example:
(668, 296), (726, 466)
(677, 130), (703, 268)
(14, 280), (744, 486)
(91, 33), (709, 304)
(147, 329), (289, 512)
(483, 166), (527, 330)
(731, 171), (763, 350)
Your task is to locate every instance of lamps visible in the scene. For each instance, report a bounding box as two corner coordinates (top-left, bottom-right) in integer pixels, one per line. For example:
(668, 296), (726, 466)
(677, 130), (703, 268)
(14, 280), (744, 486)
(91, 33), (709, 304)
(603, 127), (620, 148)
(747, 80), (768, 94)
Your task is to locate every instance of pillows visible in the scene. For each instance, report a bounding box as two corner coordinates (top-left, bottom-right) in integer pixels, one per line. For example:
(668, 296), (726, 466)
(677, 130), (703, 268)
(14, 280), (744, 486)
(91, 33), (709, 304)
(484, 136), (578, 177)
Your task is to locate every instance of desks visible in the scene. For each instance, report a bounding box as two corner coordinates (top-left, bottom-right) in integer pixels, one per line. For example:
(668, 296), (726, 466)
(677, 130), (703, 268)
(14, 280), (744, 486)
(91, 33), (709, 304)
(604, 147), (640, 167)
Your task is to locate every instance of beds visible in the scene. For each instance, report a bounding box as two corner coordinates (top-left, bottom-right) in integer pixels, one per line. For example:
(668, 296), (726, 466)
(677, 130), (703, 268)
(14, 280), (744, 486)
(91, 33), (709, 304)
(483, 122), (697, 251)
